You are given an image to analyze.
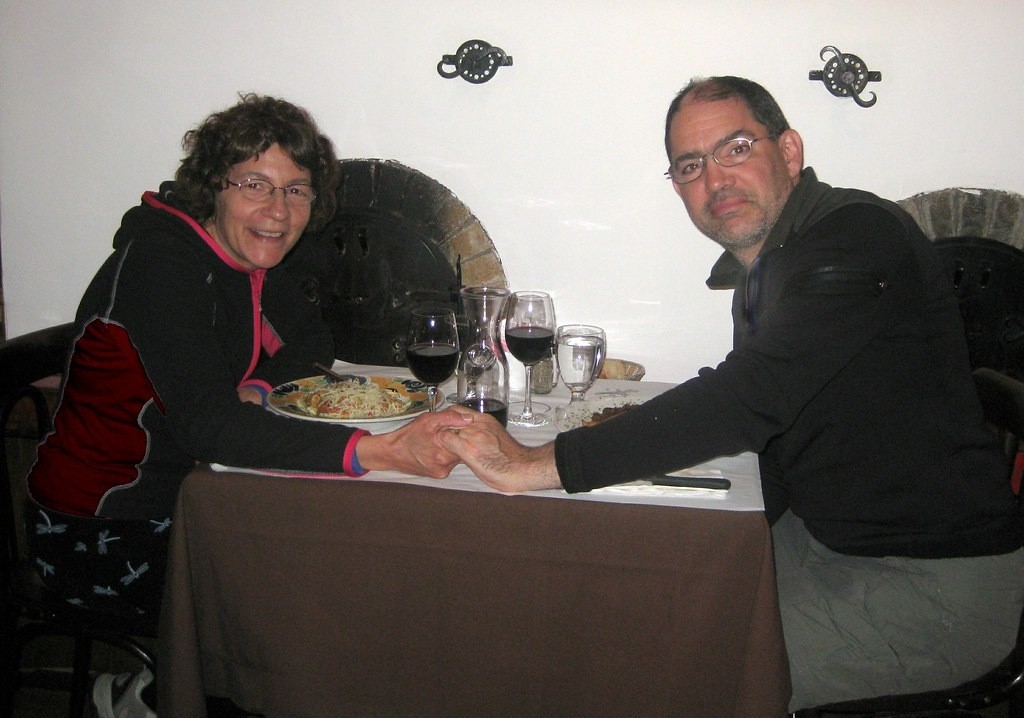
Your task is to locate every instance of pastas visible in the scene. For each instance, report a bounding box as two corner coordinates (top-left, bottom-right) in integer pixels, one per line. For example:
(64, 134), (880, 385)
(307, 379), (402, 418)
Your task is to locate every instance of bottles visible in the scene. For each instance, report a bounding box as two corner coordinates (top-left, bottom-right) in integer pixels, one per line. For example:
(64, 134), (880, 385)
(458, 286), (510, 430)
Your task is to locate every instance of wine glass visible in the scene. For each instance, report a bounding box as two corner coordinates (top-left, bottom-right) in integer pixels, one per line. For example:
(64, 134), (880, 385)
(506, 290), (549, 426)
(406, 307), (461, 413)
(554, 324), (606, 414)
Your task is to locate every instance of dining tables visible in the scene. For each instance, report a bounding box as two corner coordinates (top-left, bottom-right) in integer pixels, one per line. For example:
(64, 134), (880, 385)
(184, 379), (774, 718)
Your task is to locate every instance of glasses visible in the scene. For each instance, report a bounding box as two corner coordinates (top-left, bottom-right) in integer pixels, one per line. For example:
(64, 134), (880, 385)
(659, 129), (783, 185)
(218, 173), (319, 206)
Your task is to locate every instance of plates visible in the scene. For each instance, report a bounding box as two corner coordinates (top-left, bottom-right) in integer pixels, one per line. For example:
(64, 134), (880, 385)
(554, 394), (657, 433)
(267, 374), (445, 422)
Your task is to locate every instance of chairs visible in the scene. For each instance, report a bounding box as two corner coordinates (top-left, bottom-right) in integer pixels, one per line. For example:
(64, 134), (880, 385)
(786, 364), (1023, 718)
(0, 321), (158, 718)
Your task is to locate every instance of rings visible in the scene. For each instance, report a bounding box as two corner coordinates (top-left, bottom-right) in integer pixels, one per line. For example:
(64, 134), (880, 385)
(454, 429), (461, 436)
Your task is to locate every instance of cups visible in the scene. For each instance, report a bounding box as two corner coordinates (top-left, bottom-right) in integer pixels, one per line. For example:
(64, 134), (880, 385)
(529, 357), (552, 394)
(552, 349), (559, 388)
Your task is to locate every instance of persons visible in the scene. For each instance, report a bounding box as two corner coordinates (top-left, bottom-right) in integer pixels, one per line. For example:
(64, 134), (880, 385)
(439, 75), (1024, 712)
(18, 91), (473, 718)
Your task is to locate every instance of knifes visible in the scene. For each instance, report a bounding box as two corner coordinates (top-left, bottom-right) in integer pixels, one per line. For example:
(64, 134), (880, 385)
(614, 474), (731, 490)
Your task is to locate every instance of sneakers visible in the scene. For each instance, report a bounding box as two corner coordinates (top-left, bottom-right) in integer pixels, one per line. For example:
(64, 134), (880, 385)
(91, 667), (159, 718)
